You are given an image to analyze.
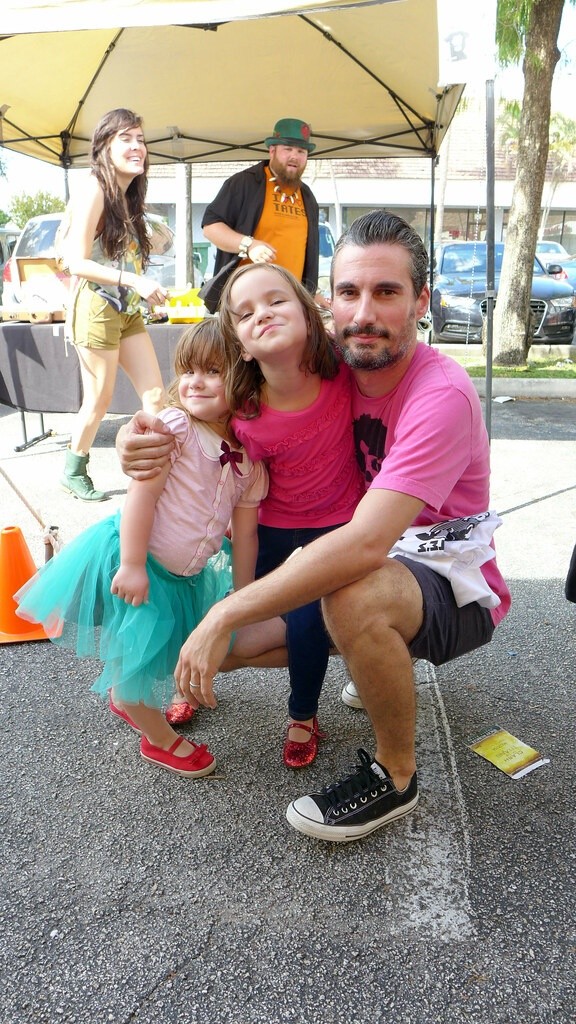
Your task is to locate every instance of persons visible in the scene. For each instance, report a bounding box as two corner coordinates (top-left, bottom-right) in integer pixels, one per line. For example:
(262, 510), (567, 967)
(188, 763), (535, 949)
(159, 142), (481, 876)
(59, 109), (171, 503)
(118, 211), (511, 843)
(13, 317), (269, 780)
(217, 264), (370, 769)
(197, 120), (330, 312)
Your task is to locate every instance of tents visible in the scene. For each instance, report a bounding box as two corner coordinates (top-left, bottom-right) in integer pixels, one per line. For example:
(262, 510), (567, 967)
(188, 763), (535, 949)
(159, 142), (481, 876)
(0, 2), (465, 452)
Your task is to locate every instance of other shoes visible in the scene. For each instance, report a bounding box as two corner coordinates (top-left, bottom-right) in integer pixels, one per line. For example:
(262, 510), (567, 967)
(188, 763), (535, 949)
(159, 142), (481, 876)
(284, 717), (319, 769)
(140, 734), (217, 778)
(109, 694), (142, 736)
(166, 702), (194, 727)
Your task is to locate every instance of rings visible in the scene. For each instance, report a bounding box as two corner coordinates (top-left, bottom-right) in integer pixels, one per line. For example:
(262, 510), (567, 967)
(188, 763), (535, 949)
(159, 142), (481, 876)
(190, 683), (199, 688)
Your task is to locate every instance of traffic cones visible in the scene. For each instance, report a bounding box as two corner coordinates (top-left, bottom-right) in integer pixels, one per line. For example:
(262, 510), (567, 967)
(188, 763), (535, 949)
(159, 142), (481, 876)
(0, 525), (65, 645)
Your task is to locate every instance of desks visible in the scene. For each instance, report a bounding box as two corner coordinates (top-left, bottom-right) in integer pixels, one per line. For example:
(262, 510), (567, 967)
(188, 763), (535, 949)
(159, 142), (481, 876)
(0, 320), (199, 451)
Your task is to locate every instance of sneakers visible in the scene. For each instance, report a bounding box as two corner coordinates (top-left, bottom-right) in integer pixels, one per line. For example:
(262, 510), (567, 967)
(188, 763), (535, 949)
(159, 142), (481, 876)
(341, 657), (422, 711)
(286, 748), (419, 841)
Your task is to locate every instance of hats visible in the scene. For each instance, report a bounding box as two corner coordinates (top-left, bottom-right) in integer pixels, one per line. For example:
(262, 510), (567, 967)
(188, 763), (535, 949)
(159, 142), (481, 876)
(265, 118), (316, 153)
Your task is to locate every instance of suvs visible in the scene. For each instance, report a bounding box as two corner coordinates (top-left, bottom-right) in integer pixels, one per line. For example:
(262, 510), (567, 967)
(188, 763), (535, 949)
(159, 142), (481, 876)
(4, 212), (207, 322)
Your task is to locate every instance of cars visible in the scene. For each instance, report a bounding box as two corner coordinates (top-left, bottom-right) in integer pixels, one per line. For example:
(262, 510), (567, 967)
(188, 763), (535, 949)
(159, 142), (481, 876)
(421, 241), (576, 346)
(534, 241), (576, 289)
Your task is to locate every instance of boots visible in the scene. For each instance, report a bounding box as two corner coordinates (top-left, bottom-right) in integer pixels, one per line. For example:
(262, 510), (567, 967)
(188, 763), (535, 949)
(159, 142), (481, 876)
(58, 443), (108, 503)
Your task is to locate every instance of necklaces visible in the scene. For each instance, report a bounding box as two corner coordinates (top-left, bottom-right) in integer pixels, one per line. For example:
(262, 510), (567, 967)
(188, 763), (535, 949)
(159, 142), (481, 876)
(267, 166), (298, 206)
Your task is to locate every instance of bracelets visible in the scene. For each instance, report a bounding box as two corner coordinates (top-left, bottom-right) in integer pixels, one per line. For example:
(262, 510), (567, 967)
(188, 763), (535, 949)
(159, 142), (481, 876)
(239, 235), (254, 260)
(119, 271), (122, 287)
